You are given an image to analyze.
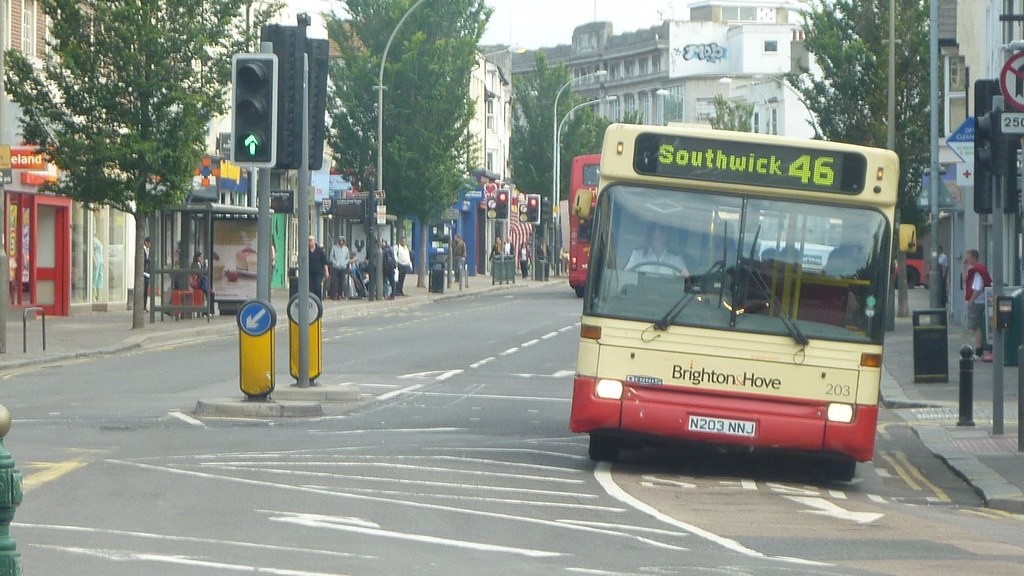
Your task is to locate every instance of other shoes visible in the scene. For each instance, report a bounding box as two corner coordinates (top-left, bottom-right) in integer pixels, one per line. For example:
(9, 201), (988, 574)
(337, 295), (343, 301)
(144, 308), (148, 312)
(971, 354), (984, 362)
(396, 292), (403, 297)
(384, 295), (395, 300)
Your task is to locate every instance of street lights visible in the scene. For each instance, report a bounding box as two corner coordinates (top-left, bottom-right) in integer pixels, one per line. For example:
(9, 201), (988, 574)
(655, 89), (702, 123)
(552, 70), (617, 267)
(718, 77), (774, 134)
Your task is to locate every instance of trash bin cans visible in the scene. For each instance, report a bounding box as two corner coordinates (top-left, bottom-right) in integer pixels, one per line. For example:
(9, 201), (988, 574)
(535, 256), (549, 280)
(429, 261), (445, 293)
(912, 309), (949, 384)
(289, 267), (311, 297)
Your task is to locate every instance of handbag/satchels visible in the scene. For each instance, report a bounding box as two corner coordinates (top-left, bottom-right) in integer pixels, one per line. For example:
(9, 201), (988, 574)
(188, 272), (203, 290)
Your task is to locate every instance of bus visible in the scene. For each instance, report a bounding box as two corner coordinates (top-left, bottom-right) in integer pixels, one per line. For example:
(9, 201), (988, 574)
(568, 120), (919, 481)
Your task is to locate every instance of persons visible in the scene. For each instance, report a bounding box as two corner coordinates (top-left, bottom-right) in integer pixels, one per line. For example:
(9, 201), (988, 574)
(170, 248), (216, 319)
(451, 235), (466, 282)
(518, 242), (531, 278)
(92, 233), (104, 303)
(489, 236), (515, 262)
(762, 245), (858, 281)
(938, 246), (949, 302)
(306, 234), (412, 301)
(142, 237), (152, 312)
(964, 251), (993, 364)
(623, 216), (690, 277)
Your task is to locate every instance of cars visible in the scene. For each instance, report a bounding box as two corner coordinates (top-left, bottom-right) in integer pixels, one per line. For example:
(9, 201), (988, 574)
(894, 244), (943, 288)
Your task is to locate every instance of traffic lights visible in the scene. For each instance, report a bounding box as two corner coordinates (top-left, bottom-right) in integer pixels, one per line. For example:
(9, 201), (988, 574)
(527, 194), (541, 225)
(974, 111), (1007, 174)
(231, 53), (277, 167)
(496, 189), (510, 219)
